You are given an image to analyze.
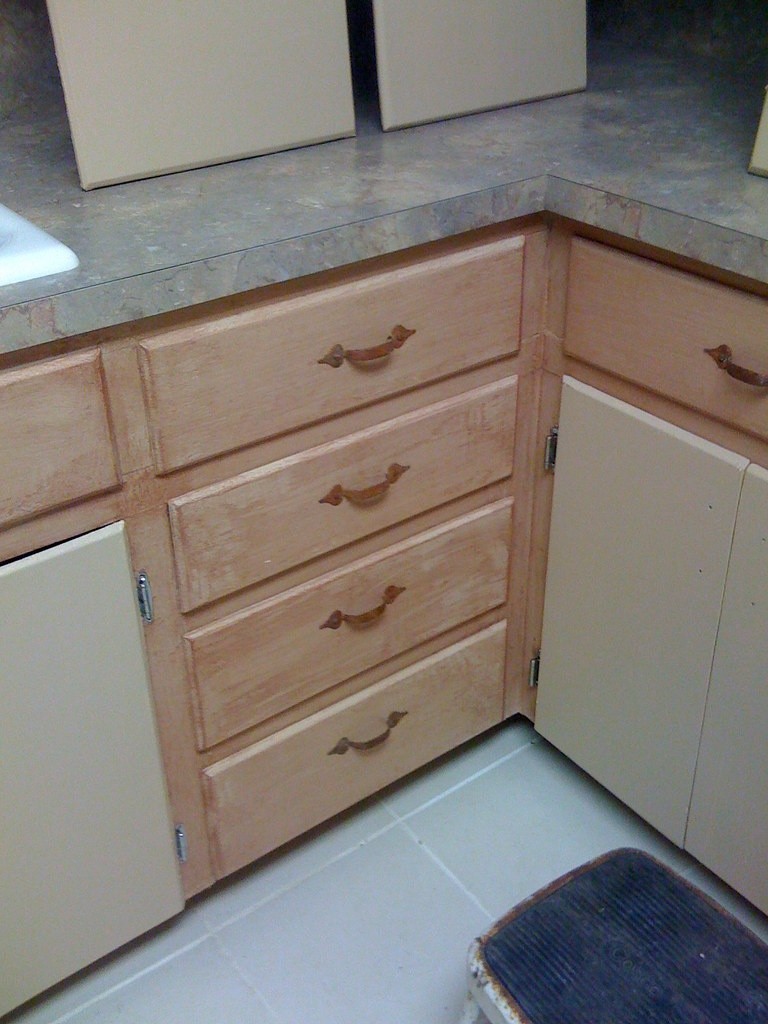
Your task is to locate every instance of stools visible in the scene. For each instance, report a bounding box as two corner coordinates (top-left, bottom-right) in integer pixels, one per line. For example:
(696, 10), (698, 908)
(461, 847), (768, 1023)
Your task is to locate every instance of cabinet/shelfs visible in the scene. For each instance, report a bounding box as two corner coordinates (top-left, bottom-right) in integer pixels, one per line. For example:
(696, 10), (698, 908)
(533, 208), (768, 924)
(142, 223), (528, 879)
(1, 342), (186, 1023)
